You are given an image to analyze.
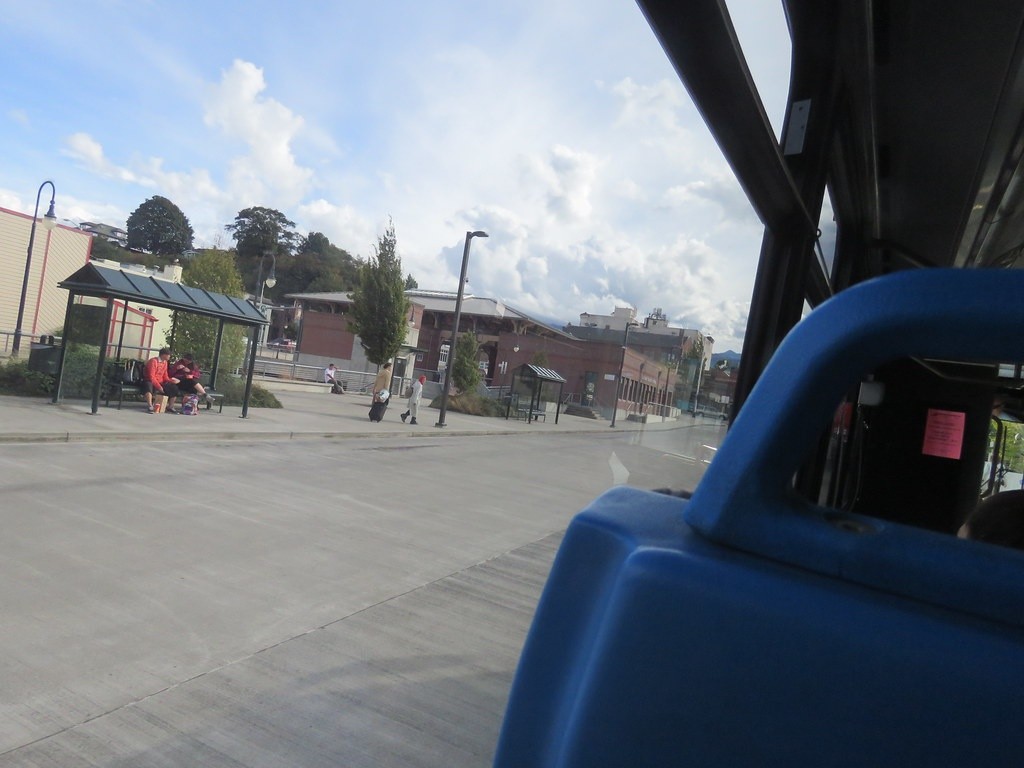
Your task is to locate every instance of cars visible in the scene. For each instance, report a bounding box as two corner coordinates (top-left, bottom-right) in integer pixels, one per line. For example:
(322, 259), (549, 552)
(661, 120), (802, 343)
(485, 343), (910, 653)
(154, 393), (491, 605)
(266, 337), (296, 353)
(242, 336), (258, 350)
(691, 406), (728, 420)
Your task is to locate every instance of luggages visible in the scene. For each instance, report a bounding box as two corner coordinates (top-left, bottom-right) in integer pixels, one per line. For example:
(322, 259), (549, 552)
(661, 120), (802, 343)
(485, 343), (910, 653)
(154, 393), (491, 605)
(368, 398), (389, 423)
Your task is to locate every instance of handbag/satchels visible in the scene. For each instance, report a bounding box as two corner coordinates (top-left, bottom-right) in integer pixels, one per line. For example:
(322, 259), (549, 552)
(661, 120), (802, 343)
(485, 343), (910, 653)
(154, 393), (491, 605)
(405, 382), (415, 399)
(375, 389), (390, 403)
(181, 394), (199, 415)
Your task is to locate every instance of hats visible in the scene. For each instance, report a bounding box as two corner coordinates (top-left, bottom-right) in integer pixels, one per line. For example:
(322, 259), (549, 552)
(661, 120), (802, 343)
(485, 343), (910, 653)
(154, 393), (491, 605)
(159, 348), (174, 354)
(419, 376), (426, 385)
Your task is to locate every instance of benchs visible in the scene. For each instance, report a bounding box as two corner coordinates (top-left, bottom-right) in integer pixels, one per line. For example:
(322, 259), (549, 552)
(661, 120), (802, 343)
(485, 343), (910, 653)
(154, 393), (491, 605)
(517, 405), (546, 423)
(105, 361), (225, 414)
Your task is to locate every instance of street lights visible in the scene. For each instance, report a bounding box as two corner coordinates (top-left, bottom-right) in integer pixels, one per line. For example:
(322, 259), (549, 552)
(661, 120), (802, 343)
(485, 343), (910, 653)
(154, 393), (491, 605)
(240, 253), (277, 380)
(435, 231), (488, 426)
(11, 181), (58, 356)
(610, 322), (639, 426)
(663, 346), (682, 423)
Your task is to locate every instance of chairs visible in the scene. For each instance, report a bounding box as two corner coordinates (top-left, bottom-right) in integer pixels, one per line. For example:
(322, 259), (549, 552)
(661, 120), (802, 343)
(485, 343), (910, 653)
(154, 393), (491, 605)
(489, 268), (1024, 768)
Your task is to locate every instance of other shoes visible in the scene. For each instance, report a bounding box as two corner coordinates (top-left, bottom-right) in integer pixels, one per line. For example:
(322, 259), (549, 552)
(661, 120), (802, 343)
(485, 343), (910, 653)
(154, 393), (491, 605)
(166, 406), (180, 414)
(148, 406), (154, 414)
(410, 417), (416, 424)
(401, 413), (407, 422)
(203, 394), (215, 404)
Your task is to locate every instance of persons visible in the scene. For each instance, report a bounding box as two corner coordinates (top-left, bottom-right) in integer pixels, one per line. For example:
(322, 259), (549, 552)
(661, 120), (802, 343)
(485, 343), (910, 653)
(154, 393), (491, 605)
(983, 455), (1005, 494)
(169, 353), (215, 403)
(324, 364), (343, 393)
(401, 376), (426, 424)
(142, 348), (180, 414)
(957, 489), (1024, 550)
(373, 362), (393, 406)
